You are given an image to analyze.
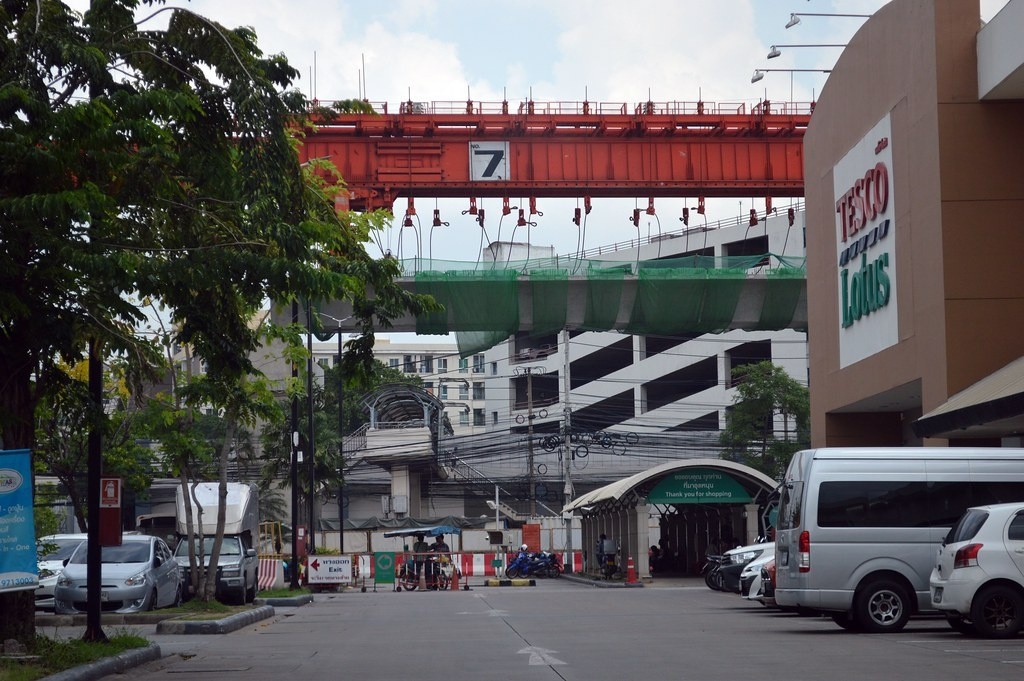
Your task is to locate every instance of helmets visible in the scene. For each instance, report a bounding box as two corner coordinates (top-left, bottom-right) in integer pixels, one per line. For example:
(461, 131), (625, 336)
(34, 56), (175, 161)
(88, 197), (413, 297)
(521, 544), (527, 551)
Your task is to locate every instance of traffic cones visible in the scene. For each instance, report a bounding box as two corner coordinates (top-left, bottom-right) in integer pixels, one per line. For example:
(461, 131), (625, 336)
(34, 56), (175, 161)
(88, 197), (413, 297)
(450, 565), (460, 591)
(627, 556), (639, 583)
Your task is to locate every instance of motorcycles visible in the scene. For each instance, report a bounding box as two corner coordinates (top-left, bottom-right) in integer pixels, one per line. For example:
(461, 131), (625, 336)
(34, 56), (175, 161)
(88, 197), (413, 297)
(398, 547), (448, 591)
(505, 544), (563, 579)
(595, 541), (618, 582)
(701, 535), (766, 593)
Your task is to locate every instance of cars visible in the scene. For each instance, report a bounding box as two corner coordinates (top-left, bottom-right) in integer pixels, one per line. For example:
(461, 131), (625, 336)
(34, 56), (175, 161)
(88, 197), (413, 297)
(719, 541), (776, 595)
(761, 558), (776, 604)
(930, 502), (1024, 637)
(35, 533), (88, 614)
(53, 534), (182, 615)
(739, 546), (775, 608)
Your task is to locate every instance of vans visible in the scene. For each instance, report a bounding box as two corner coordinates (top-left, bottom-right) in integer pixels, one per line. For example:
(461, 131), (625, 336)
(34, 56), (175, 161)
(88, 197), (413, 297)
(766, 447), (1024, 633)
(137, 514), (178, 552)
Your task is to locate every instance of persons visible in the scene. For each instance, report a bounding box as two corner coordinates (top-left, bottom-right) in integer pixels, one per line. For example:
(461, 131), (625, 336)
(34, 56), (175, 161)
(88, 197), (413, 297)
(596, 534), (611, 567)
(429, 534), (450, 590)
(413, 535), (431, 590)
(647, 535), (741, 576)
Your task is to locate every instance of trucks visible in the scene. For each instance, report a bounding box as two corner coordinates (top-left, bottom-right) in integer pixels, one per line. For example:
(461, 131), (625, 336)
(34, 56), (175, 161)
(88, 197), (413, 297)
(173, 482), (257, 607)
